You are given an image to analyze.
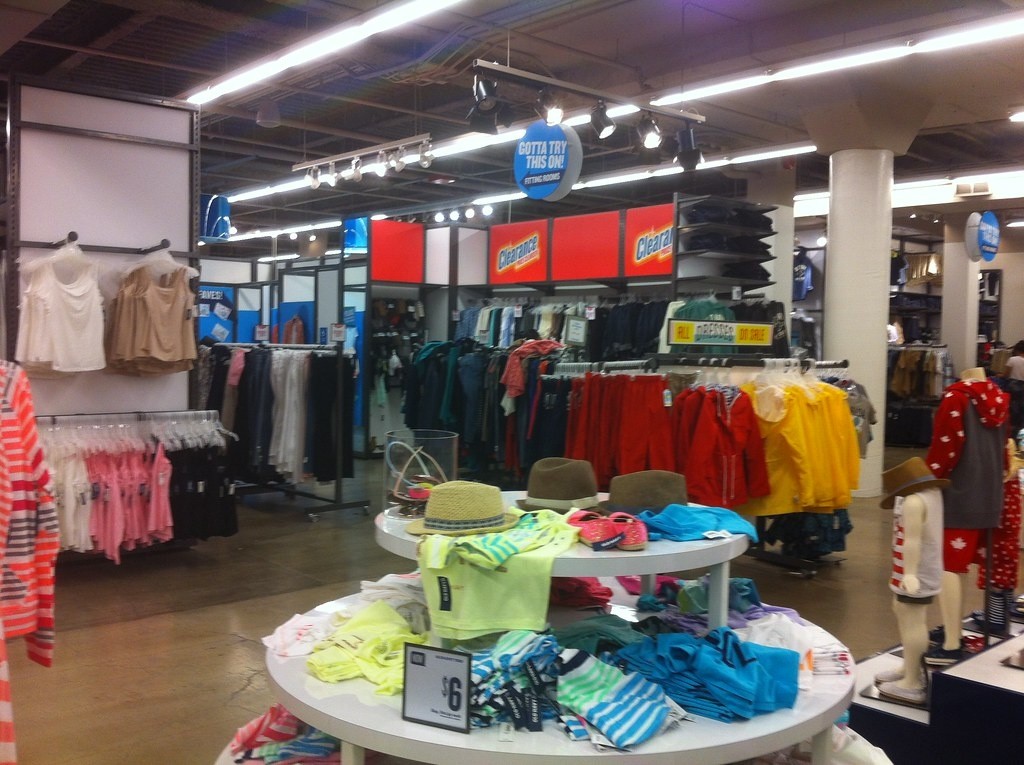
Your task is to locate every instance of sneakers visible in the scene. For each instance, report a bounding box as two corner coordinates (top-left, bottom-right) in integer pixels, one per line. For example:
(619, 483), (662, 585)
(566, 510), (647, 551)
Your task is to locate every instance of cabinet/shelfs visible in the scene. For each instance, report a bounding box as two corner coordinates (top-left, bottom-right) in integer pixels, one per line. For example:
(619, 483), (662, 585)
(209, 490), (879, 765)
(672, 193), (779, 305)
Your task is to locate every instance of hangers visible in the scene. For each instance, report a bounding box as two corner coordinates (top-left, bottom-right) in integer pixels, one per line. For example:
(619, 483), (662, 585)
(21, 233), (99, 272)
(540, 359), (665, 382)
(680, 357), (849, 394)
(476, 291), (670, 307)
(888, 342), (950, 356)
(35, 409), (240, 456)
(115, 246), (200, 279)
(233, 345), (353, 358)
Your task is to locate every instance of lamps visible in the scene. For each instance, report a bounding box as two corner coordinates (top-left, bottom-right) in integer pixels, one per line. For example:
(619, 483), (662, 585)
(464, 74), (516, 135)
(256, 98), (282, 128)
(389, 146), (407, 172)
(533, 87), (564, 127)
(673, 120), (704, 170)
(636, 111), (663, 149)
(591, 100), (616, 139)
(419, 144), (431, 167)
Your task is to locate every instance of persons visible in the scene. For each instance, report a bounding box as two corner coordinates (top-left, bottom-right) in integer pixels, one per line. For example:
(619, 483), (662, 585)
(997, 340), (1024, 452)
(924, 369), (1024, 656)
(875, 457), (951, 703)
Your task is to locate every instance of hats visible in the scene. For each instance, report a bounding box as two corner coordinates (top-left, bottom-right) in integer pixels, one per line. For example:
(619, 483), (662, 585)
(405, 480), (520, 536)
(878, 456), (950, 509)
(581, 470), (688, 521)
(515, 457), (599, 514)
